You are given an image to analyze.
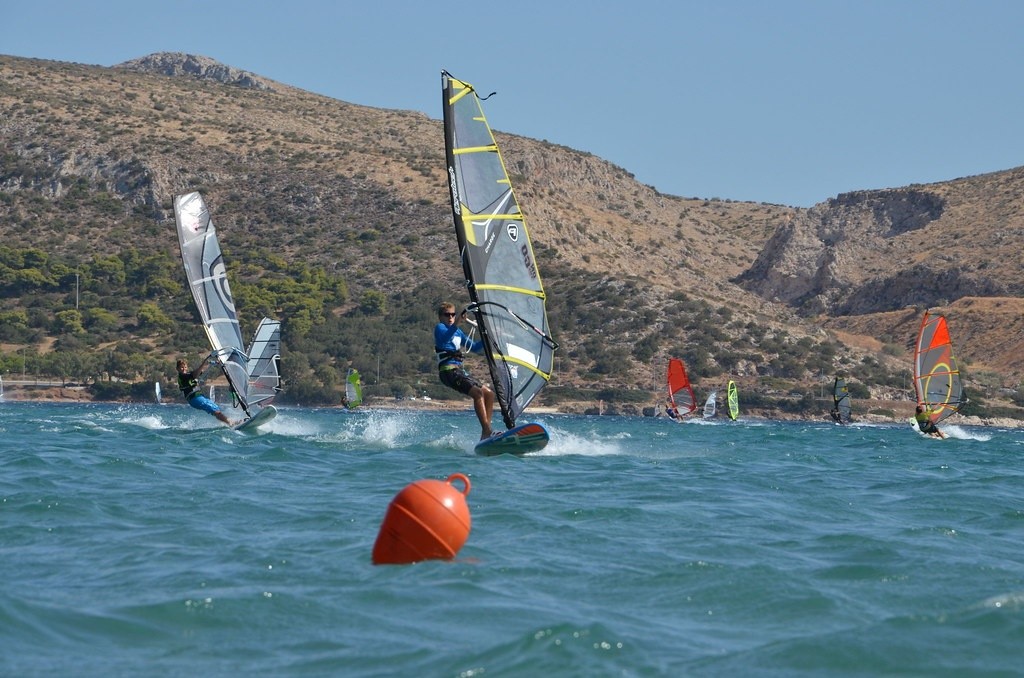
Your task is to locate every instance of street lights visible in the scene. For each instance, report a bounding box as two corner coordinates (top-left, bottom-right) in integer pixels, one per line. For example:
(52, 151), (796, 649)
(74, 273), (79, 313)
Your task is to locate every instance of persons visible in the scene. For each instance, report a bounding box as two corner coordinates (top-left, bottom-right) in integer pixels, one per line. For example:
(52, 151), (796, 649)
(701, 407), (705, 418)
(434, 301), (504, 442)
(666, 404), (682, 421)
(341, 396), (352, 410)
(831, 408), (845, 425)
(176, 359), (243, 428)
(726, 408), (737, 421)
(915, 405), (944, 438)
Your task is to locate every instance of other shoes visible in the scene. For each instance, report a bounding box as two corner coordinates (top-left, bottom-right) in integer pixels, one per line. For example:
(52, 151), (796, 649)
(229, 420), (242, 428)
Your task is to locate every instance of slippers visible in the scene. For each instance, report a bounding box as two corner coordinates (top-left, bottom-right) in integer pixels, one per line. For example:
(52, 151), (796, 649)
(490, 430), (504, 437)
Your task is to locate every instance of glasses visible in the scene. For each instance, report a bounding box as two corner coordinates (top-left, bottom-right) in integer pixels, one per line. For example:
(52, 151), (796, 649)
(442, 313), (456, 317)
